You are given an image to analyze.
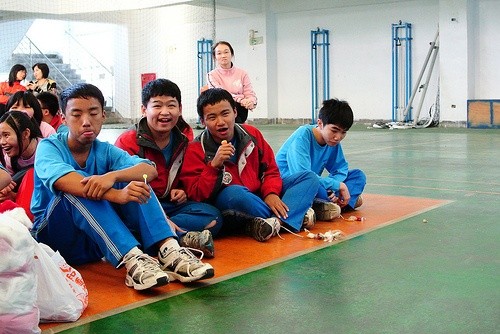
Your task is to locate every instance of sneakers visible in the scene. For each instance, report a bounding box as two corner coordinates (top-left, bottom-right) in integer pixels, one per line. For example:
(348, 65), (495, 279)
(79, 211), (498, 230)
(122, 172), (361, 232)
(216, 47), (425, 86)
(314, 202), (341, 220)
(355, 196), (363, 209)
(124, 253), (170, 291)
(304, 208), (316, 229)
(250, 217), (281, 242)
(158, 246), (215, 282)
(181, 230), (214, 258)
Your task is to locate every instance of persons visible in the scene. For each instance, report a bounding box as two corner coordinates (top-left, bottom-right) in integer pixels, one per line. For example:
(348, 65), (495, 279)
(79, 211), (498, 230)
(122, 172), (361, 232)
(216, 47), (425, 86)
(0, 62), (366, 291)
(204, 40), (258, 123)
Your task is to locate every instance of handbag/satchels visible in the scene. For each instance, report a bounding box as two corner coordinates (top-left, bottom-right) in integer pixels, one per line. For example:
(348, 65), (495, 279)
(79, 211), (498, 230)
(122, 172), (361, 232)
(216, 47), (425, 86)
(33, 236), (88, 321)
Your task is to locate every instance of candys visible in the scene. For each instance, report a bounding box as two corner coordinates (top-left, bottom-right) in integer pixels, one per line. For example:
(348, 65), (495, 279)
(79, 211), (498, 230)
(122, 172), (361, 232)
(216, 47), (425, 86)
(221, 140), (227, 144)
(143, 174), (147, 184)
